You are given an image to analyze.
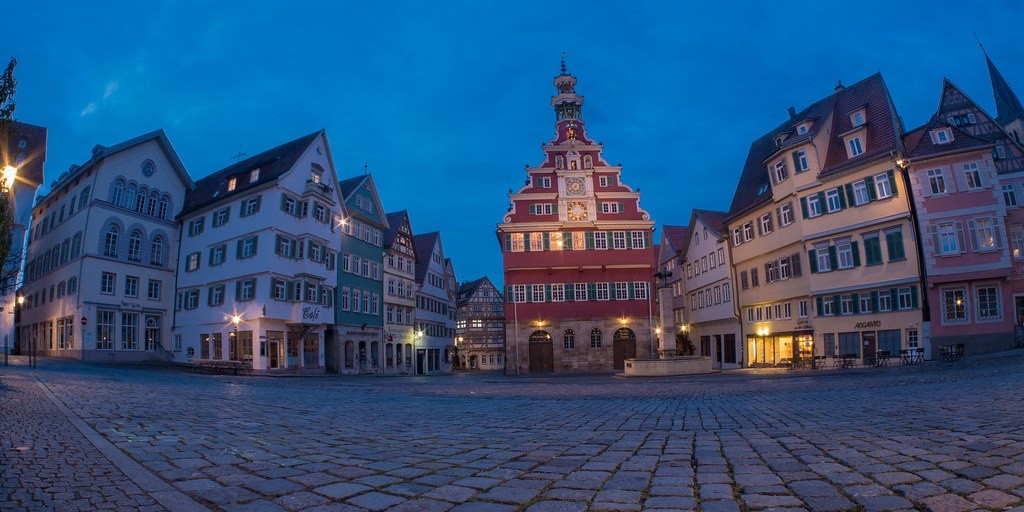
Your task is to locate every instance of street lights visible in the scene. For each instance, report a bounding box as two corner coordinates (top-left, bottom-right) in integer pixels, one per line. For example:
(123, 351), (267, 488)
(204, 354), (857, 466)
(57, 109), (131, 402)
(233, 316), (242, 372)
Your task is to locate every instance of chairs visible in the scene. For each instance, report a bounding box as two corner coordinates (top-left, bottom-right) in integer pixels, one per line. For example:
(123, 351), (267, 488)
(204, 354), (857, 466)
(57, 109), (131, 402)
(817, 356), (827, 370)
(813, 356), (821, 369)
(877, 351), (892, 367)
(952, 343), (965, 361)
(845, 353), (857, 369)
(913, 348), (925, 365)
(898, 350), (911, 366)
(832, 355), (841, 369)
(946, 344), (956, 362)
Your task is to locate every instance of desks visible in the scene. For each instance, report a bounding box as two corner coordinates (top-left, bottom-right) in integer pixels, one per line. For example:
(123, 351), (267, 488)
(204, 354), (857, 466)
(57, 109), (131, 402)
(904, 349), (917, 365)
(838, 354), (848, 369)
